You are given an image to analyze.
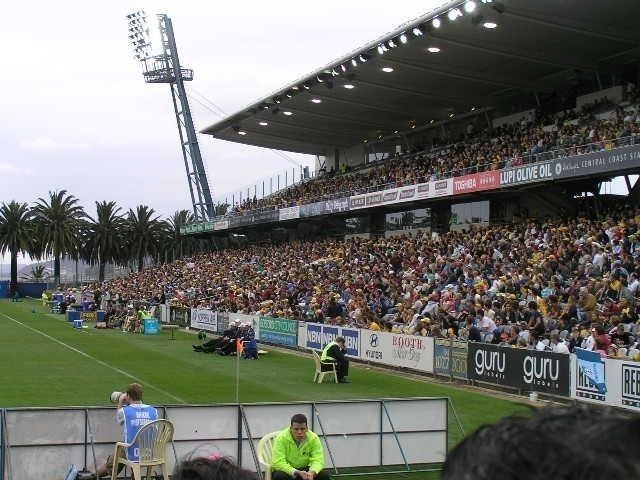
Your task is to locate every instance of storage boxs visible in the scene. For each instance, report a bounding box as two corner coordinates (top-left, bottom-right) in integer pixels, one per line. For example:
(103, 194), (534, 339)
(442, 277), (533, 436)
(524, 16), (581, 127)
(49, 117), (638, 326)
(74, 319), (83, 328)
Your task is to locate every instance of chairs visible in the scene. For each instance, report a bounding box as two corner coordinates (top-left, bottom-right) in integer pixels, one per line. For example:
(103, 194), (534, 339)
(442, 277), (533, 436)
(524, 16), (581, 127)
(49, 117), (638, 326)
(111, 418), (175, 480)
(256, 429), (284, 479)
(311, 349), (339, 384)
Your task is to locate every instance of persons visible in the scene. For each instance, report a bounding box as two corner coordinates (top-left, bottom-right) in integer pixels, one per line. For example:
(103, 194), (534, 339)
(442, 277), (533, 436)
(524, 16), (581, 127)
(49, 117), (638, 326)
(320, 337), (350, 383)
(270, 414), (330, 480)
(439, 398), (640, 479)
(42, 202), (639, 359)
(232, 78), (640, 213)
(78, 383), (158, 478)
(171, 442), (258, 480)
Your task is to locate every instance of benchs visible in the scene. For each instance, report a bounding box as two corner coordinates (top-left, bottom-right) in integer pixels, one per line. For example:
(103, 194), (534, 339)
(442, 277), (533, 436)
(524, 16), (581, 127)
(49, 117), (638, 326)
(237, 339), (252, 357)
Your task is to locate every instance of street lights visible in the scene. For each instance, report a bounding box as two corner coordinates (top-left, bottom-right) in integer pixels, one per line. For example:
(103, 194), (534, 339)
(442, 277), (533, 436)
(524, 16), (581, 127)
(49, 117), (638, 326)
(126, 7), (217, 225)
